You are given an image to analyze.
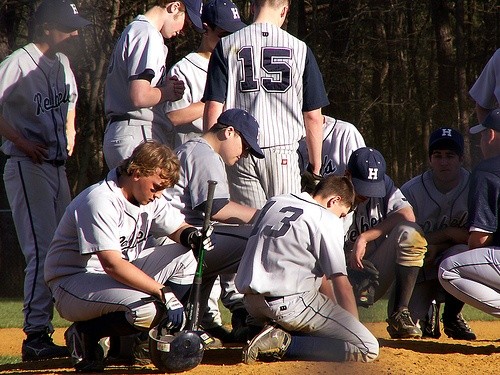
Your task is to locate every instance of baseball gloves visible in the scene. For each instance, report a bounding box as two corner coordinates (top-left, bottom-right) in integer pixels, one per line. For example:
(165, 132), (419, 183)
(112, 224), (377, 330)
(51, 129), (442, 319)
(348, 260), (380, 307)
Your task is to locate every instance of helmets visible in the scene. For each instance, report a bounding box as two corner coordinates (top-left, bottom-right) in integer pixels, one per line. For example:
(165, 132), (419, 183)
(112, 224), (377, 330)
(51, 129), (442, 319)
(149, 324), (204, 372)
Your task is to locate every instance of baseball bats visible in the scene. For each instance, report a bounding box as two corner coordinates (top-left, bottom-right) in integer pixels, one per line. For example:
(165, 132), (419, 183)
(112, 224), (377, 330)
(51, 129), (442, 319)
(186, 181), (217, 333)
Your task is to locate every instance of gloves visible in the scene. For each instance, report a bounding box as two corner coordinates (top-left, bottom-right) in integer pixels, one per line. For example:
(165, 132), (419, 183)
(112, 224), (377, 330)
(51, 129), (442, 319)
(180, 227), (217, 251)
(140, 285), (187, 331)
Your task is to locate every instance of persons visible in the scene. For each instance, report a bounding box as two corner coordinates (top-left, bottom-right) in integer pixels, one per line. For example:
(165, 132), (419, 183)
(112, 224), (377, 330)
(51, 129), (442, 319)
(235, 148), (428, 364)
(0, 0), (93, 365)
(387, 48), (500, 340)
(44, 140), (217, 372)
(160, 108), (266, 347)
(102, 0), (367, 210)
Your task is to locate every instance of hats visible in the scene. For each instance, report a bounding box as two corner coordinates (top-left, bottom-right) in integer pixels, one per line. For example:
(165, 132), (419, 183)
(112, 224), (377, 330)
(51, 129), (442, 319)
(429, 126), (464, 153)
(469, 110), (500, 137)
(348, 147), (387, 197)
(184, 0), (203, 30)
(36, 0), (93, 29)
(206, 0), (247, 33)
(218, 108), (265, 159)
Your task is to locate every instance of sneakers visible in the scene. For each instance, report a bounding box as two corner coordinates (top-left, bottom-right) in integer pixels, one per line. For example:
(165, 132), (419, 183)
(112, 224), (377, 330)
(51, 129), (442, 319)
(232, 316), (265, 341)
(22, 339), (72, 361)
(242, 324), (290, 365)
(107, 340), (153, 364)
(415, 299), (441, 339)
(442, 313), (476, 341)
(198, 321), (236, 343)
(64, 323), (91, 370)
(188, 328), (222, 348)
(386, 310), (422, 338)
